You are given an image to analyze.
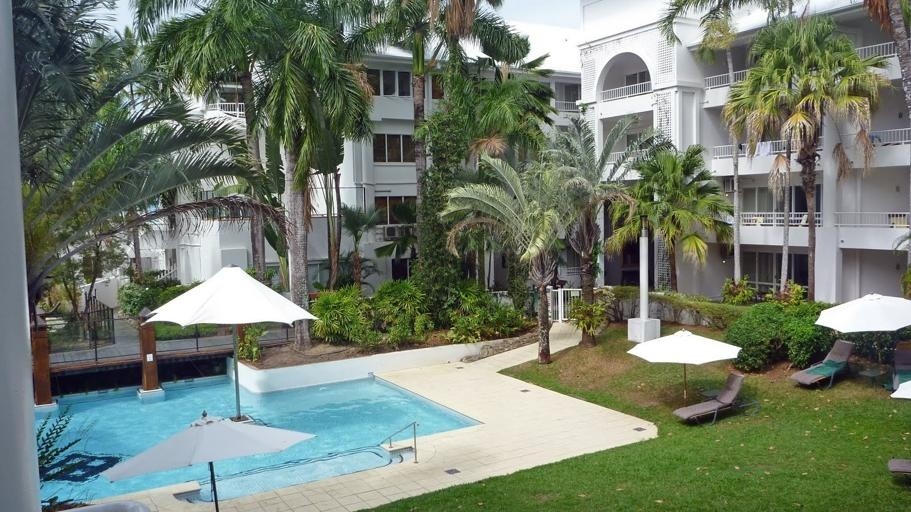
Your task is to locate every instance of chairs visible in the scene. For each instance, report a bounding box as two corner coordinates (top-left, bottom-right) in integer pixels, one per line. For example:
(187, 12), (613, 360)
(672, 371), (746, 428)
(890, 339), (911, 390)
(789, 337), (856, 390)
(887, 458), (911, 477)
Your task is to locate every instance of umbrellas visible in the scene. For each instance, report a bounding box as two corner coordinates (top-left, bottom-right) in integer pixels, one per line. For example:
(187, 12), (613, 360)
(141, 267), (316, 417)
(100, 418), (316, 512)
(813, 294), (911, 364)
(627, 329), (743, 402)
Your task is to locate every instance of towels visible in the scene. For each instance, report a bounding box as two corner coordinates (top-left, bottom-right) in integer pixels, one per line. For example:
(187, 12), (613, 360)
(805, 360), (849, 377)
(898, 369), (911, 384)
(879, 382), (893, 391)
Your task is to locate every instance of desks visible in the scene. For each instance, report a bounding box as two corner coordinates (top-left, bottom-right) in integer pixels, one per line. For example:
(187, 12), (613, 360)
(857, 367), (888, 392)
(699, 389), (721, 400)
